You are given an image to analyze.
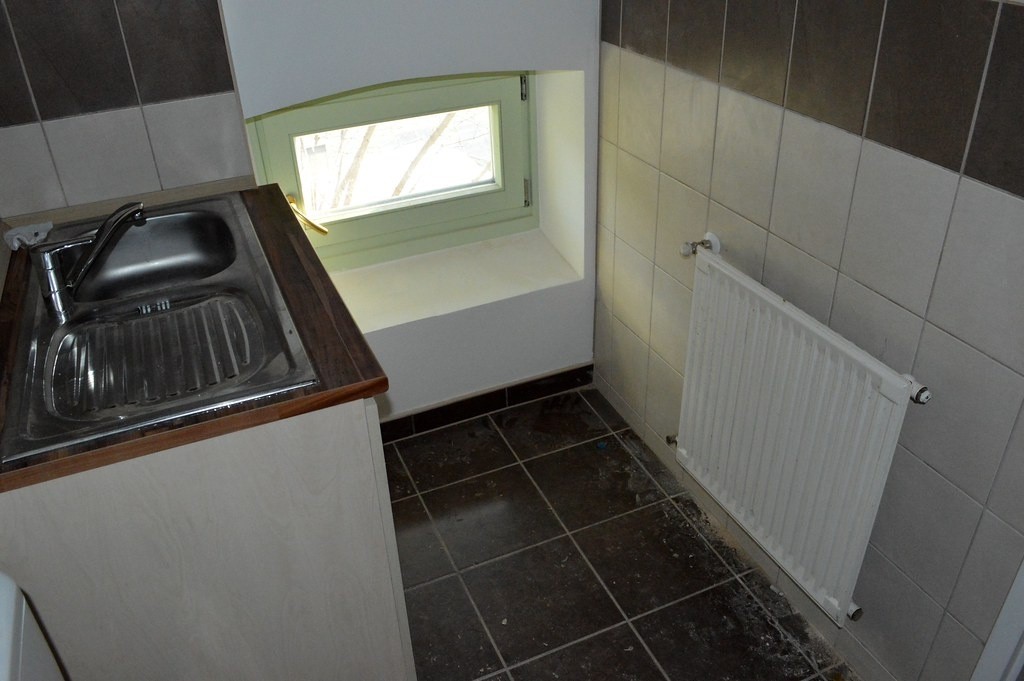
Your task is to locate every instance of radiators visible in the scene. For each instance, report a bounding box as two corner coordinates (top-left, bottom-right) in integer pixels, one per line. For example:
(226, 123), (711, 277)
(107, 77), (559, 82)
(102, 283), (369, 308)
(673, 244), (910, 629)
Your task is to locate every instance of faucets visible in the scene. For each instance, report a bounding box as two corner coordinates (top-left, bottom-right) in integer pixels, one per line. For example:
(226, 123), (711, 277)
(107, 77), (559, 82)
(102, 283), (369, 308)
(28, 201), (148, 340)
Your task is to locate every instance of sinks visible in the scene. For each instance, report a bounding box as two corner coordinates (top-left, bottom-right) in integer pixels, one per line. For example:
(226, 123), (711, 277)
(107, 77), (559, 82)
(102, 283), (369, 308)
(0, 190), (273, 315)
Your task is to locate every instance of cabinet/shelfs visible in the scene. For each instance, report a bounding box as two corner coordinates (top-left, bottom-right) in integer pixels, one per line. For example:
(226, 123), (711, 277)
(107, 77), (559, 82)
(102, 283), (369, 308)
(0, 398), (419, 681)
